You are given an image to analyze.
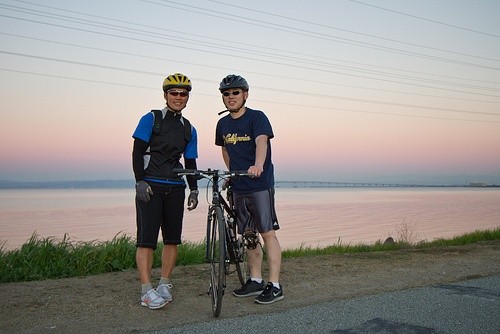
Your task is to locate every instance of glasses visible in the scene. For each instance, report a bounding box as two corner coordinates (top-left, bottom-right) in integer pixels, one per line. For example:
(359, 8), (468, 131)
(224, 91), (240, 96)
(167, 90), (188, 97)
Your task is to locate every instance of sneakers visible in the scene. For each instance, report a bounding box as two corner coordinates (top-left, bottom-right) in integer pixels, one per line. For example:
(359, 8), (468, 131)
(140, 288), (167, 309)
(254, 282), (284, 305)
(156, 284), (173, 302)
(233, 278), (265, 298)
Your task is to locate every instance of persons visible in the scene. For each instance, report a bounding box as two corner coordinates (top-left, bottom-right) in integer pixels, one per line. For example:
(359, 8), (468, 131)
(130, 73), (200, 309)
(214, 74), (285, 305)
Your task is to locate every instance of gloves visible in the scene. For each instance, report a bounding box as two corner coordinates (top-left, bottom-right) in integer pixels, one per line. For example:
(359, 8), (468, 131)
(135, 181), (153, 203)
(187, 190), (199, 211)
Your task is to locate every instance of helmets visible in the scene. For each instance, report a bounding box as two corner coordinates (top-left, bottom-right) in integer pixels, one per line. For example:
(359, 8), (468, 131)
(219, 74), (249, 92)
(163, 73), (192, 93)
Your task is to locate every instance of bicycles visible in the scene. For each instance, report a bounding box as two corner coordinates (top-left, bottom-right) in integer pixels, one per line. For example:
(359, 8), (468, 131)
(169, 165), (266, 318)
